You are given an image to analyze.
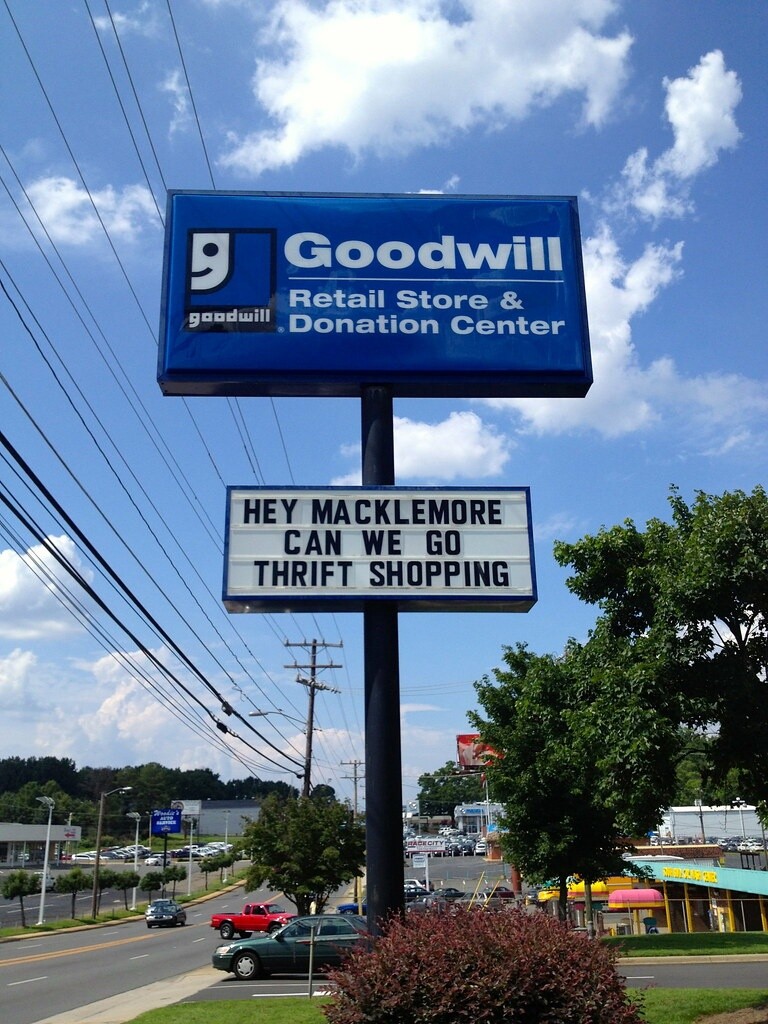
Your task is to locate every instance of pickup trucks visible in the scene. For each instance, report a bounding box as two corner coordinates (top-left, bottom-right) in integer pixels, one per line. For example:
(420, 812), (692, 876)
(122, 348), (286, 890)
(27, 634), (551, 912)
(209, 902), (298, 939)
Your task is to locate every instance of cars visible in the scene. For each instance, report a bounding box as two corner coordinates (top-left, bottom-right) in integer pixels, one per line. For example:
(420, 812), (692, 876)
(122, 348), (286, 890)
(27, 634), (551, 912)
(146, 842), (233, 866)
(403, 826), (487, 858)
(336, 897), (367, 916)
(649, 833), (765, 852)
(145, 899), (186, 929)
(404, 879), (515, 910)
(62, 843), (151, 862)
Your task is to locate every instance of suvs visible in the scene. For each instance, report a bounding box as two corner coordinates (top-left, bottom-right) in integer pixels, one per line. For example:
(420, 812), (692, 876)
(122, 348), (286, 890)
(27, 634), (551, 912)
(454, 890), (504, 911)
(211, 914), (368, 981)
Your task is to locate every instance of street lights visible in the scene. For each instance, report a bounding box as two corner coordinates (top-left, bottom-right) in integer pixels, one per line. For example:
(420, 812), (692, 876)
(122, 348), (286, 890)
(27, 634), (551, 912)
(126, 813), (141, 910)
(249, 710), (314, 803)
(35, 796), (56, 925)
(732, 796), (748, 852)
(91, 786), (133, 917)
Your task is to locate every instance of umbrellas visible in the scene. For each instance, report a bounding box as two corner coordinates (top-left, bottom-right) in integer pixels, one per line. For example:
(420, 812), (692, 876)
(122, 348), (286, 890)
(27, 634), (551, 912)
(567, 877), (609, 897)
(608, 885), (665, 935)
(538, 883), (575, 901)
